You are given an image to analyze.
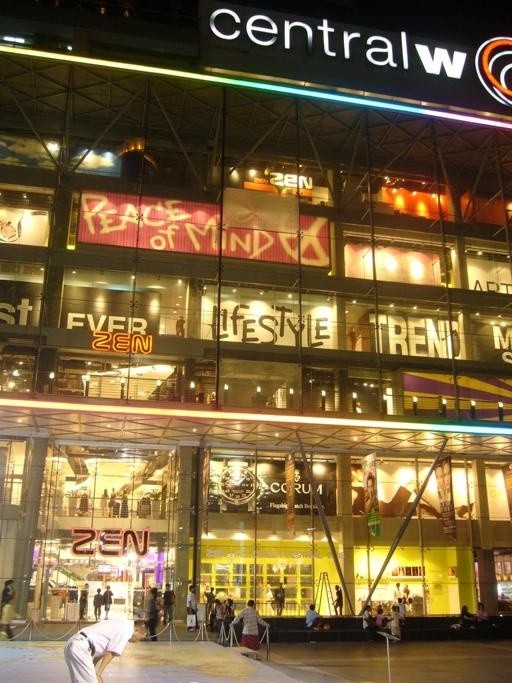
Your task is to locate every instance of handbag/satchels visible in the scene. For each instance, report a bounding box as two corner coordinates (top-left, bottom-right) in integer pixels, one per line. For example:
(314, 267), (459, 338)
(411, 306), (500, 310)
(186, 611), (197, 627)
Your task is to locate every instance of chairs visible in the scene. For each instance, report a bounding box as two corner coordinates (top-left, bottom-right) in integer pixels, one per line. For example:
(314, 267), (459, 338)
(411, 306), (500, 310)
(378, 486), (412, 516)
(413, 484), (473, 518)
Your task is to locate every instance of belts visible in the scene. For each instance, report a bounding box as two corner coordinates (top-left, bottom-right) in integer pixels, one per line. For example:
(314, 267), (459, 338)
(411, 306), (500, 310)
(78, 631), (96, 657)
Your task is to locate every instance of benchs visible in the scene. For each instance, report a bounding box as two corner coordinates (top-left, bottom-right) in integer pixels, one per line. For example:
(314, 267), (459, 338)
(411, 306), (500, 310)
(224, 615), (512, 642)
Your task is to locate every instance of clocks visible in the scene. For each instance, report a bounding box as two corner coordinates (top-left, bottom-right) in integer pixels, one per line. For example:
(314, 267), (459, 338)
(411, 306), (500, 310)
(218, 465), (259, 505)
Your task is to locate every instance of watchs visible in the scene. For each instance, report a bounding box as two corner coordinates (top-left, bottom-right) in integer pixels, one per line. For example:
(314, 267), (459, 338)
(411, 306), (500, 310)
(217, 457), (263, 514)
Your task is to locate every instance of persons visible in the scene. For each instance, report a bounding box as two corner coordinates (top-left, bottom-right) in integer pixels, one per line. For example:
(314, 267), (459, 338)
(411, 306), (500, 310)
(120, 489), (130, 518)
(64, 616), (149, 683)
(362, 583), (408, 644)
(78, 583), (113, 621)
(475, 601), (489, 626)
(305, 605), (322, 631)
(273, 583), (285, 617)
(459, 604), (476, 629)
(365, 472), (378, 514)
(1, 579), (17, 641)
(176, 314), (186, 337)
(143, 583), (176, 641)
(101, 488), (109, 515)
(434, 465), (444, 496)
(109, 487), (117, 517)
(442, 462), (450, 488)
(186, 585), (270, 651)
(348, 326), (357, 349)
(332, 585), (344, 617)
